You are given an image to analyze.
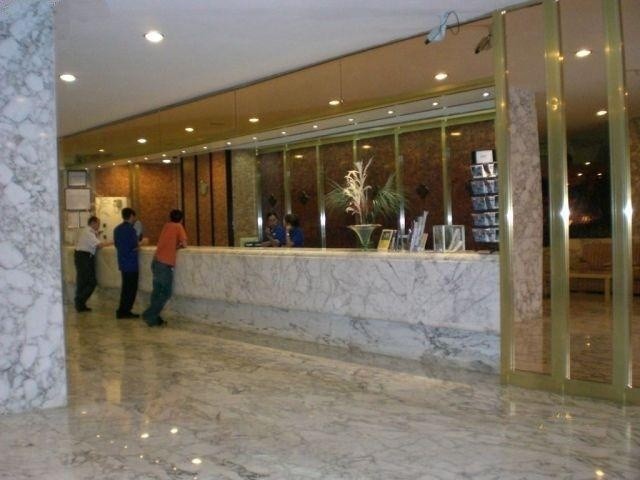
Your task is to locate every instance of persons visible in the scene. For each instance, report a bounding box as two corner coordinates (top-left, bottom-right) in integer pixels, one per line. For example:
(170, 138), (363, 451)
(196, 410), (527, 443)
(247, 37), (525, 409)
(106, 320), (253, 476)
(113, 208), (149, 318)
(265, 214), (304, 247)
(74, 216), (115, 312)
(262, 212), (284, 246)
(143, 209), (187, 327)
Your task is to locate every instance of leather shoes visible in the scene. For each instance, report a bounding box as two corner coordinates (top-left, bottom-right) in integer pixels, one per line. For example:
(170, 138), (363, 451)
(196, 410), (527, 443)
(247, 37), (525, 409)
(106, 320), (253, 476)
(74, 296), (92, 312)
(116, 310), (140, 319)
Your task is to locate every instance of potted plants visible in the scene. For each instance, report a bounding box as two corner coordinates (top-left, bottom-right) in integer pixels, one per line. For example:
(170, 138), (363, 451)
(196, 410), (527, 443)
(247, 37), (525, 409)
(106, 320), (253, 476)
(322, 156), (414, 251)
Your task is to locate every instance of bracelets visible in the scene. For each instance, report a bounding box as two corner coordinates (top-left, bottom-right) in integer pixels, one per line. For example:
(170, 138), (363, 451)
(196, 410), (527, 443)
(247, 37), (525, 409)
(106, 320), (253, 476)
(286, 232), (290, 236)
